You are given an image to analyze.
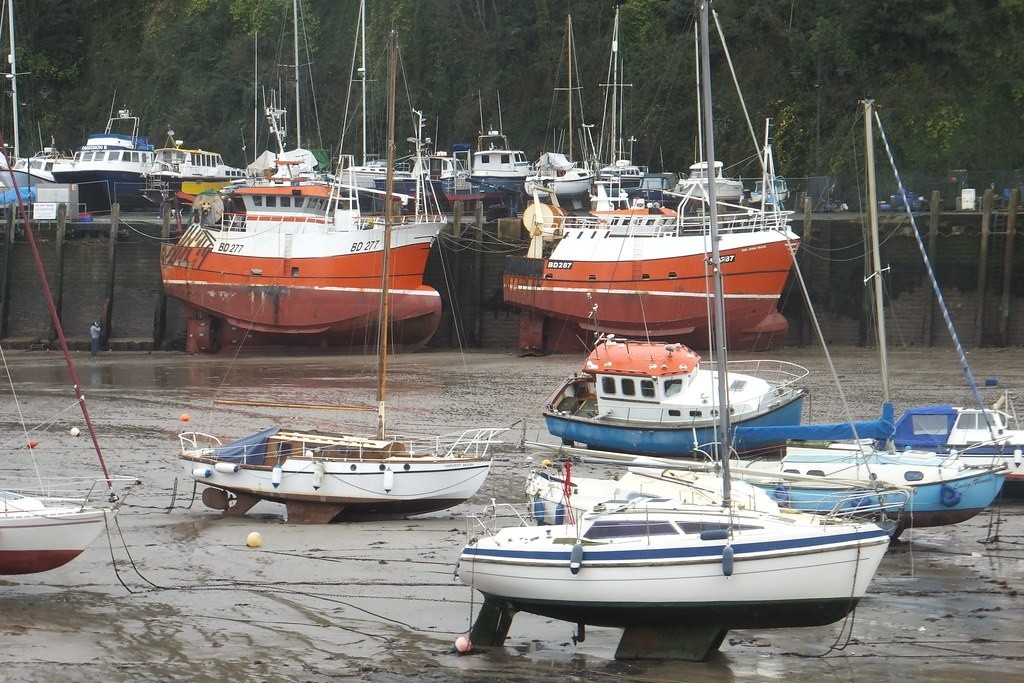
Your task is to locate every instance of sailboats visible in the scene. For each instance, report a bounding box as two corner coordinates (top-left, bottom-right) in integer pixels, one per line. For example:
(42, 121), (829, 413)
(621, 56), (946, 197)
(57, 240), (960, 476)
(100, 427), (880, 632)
(0, 140), (142, 579)
(178, 25), (512, 521)
(159, 1), (800, 357)
(522, 98), (1014, 541)
(451, 0), (911, 662)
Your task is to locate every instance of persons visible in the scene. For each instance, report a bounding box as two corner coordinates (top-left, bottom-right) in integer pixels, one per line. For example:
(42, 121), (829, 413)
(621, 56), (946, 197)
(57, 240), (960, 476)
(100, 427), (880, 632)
(90, 322), (101, 355)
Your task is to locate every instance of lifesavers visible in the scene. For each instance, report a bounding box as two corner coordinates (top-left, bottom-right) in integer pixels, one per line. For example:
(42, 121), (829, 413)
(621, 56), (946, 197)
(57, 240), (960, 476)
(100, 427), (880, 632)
(852, 488), (870, 506)
(941, 484), (961, 508)
(771, 486), (788, 506)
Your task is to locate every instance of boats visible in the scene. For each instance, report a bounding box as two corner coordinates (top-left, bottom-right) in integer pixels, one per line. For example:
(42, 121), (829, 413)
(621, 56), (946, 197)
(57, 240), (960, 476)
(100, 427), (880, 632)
(825, 388), (1024, 481)
(0, 89), (246, 218)
(543, 291), (812, 458)
